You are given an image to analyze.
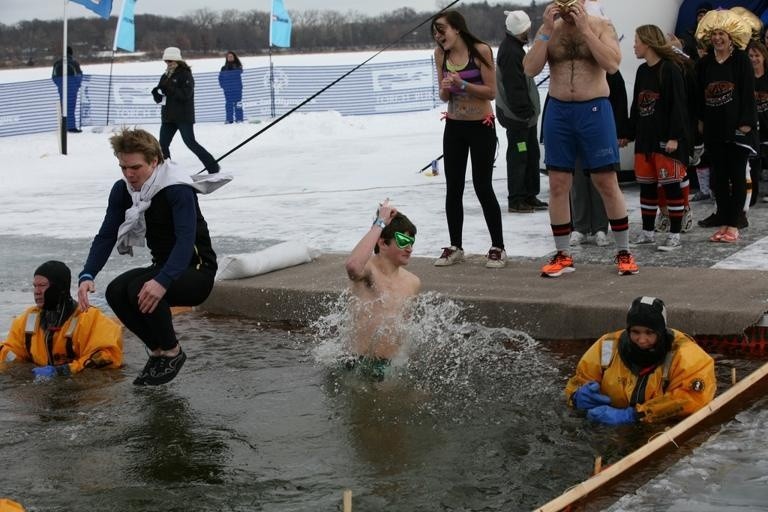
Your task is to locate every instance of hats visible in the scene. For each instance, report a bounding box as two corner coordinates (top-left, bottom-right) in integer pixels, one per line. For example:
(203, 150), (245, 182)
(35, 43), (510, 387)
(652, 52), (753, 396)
(503, 8), (532, 36)
(626, 295), (668, 337)
(160, 45), (183, 63)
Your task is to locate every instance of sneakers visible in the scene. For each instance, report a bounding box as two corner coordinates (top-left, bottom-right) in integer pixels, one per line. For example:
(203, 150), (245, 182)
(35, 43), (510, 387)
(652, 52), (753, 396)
(593, 229), (611, 248)
(656, 235), (685, 253)
(433, 245), (465, 268)
(506, 195), (550, 215)
(629, 231), (656, 247)
(566, 230), (590, 245)
(615, 249), (642, 277)
(485, 245), (511, 269)
(539, 250), (577, 278)
(130, 346), (186, 388)
(688, 187), (768, 243)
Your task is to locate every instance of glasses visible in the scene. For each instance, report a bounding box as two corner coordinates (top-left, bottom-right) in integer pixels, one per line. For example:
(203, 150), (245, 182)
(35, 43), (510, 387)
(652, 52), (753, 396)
(391, 231), (416, 250)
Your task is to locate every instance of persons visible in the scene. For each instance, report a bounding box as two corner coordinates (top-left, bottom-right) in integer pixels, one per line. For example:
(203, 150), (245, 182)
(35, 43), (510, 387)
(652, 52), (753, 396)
(565, 296), (716, 454)
(0, 261), (123, 386)
(151, 47), (220, 174)
(431, 11), (507, 268)
(52, 46), (83, 133)
(219, 51), (244, 124)
(343, 196), (422, 383)
(77, 130), (216, 386)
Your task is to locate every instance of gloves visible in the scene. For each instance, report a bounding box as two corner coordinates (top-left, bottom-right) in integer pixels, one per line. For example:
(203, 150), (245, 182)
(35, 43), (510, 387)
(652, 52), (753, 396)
(572, 380), (612, 412)
(584, 404), (637, 427)
(30, 363), (74, 384)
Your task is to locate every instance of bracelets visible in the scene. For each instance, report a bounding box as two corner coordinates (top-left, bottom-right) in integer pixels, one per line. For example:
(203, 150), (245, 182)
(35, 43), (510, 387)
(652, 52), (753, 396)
(460, 80), (467, 91)
(78, 274), (93, 280)
(375, 219), (386, 229)
